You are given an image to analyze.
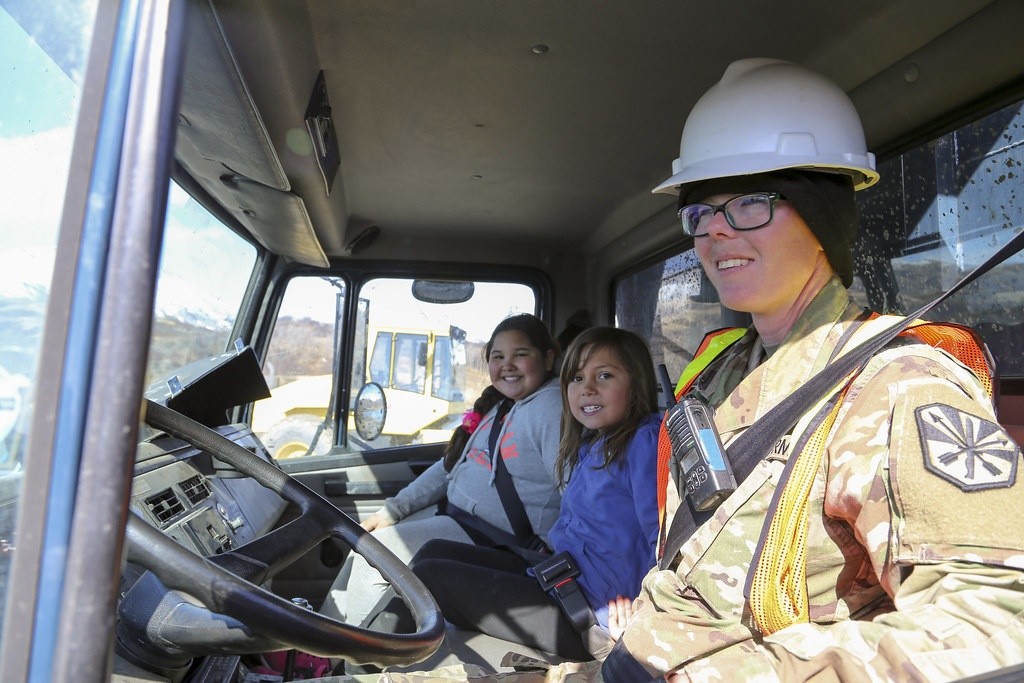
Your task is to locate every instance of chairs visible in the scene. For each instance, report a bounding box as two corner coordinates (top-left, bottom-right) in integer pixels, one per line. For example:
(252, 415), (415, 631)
(344, 600), (566, 675)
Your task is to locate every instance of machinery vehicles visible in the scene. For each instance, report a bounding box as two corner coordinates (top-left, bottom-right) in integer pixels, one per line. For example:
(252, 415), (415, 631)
(254, 320), (474, 465)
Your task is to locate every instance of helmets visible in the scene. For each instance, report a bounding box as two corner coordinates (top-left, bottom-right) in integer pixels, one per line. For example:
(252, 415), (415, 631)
(652, 57), (880, 196)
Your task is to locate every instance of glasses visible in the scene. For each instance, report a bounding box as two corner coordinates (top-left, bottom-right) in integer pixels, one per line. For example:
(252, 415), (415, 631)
(678, 192), (788, 237)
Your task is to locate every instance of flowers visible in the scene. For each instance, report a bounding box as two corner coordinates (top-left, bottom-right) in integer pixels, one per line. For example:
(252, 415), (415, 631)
(461, 411), (480, 434)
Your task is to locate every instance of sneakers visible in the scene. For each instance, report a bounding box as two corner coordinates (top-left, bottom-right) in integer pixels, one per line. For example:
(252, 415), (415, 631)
(259, 648), (331, 673)
(252, 667), (305, 678)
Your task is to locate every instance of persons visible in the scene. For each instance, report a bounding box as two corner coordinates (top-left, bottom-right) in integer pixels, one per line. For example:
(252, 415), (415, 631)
(241, 57), (1024, 682)
